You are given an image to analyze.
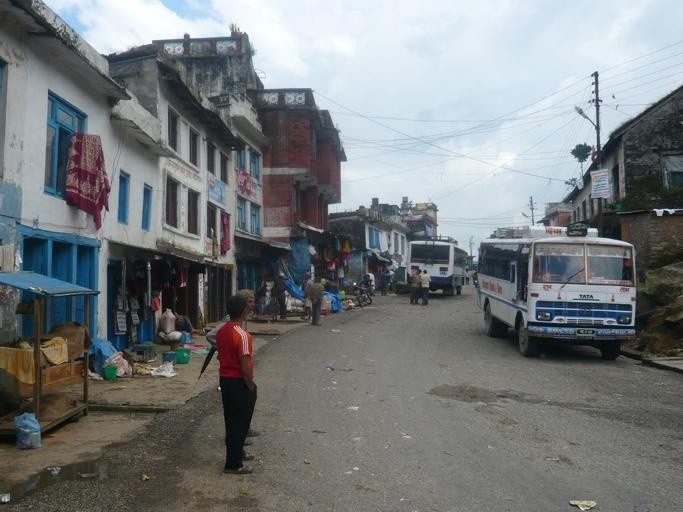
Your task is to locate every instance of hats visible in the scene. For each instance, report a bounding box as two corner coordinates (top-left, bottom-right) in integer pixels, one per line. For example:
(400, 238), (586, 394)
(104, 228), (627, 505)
(238, 289), (256, 300)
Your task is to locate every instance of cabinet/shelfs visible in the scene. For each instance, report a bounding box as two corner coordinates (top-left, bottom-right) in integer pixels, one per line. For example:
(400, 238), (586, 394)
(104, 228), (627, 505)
(0, 272), (100, 436)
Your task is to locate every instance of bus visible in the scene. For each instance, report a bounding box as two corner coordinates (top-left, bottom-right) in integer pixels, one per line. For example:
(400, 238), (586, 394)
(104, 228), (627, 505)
(407, 236), (468, 296)
(475, 228), (646, 359)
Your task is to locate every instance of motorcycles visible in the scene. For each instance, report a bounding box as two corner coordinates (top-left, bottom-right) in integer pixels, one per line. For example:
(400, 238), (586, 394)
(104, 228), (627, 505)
(352, 282), (373, 306)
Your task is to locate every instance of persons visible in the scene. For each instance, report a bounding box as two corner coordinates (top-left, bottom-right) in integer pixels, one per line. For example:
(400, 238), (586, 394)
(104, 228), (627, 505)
(206, 288), (260, 446)
(257, 272), (324, 326)
(465, 269), (470, 285)
(379, 268), (432, 305)
(215, 295), (258, 475)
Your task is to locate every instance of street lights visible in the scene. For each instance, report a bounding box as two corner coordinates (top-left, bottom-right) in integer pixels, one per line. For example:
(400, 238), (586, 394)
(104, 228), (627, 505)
(574, 105), (604, 237)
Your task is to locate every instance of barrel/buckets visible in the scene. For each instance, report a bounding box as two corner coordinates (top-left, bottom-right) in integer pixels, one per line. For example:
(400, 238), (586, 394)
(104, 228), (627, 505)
(162, 351), (176, 363)
(176, 348), (191, 364)
(102, 362), (118, 380)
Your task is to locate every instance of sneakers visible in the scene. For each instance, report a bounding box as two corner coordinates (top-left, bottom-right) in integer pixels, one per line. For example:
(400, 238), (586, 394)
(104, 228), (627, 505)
(223, 428), (260, 474)
